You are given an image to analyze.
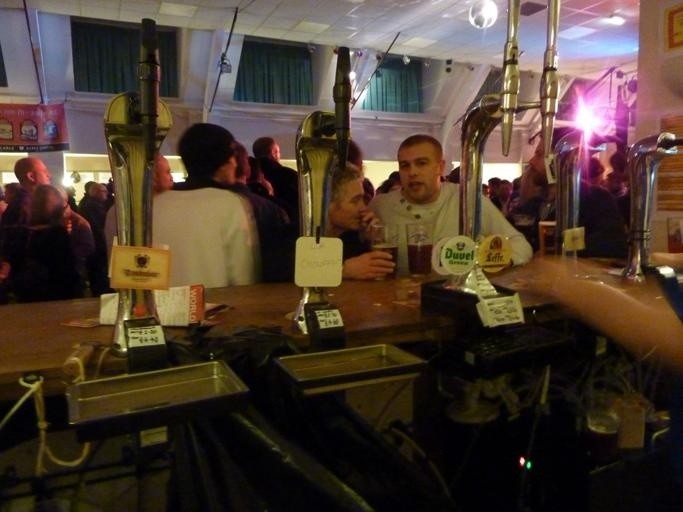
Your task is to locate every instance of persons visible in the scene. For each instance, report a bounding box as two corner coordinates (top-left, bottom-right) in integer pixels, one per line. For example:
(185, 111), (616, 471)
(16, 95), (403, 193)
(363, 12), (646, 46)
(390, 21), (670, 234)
(520, 246), (682, 511)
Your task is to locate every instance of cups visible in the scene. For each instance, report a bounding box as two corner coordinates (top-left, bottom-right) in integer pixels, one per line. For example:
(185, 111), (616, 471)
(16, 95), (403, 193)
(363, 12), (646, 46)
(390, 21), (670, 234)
(587, 375), (630, 434)
(405, 222), (436, 276)
(371, 222), (400, 280)
